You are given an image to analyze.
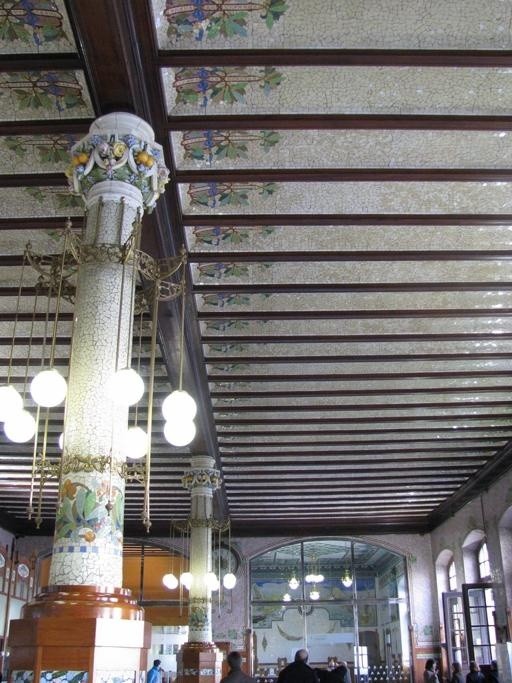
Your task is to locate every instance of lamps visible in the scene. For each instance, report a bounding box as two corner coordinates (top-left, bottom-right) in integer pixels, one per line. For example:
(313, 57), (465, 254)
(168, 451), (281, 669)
(281, 541), (355, 603)
(0, 194), (196, 536)
(162, 514), (236, 620)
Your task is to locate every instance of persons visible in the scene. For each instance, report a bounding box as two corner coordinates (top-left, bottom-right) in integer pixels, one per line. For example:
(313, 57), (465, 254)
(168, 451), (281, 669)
(277, 650), (319, 683)
(451, 663), (465, 683)
(466, 661), (486, 683)
(424, 659), (440, 683)
(147, 660), (161, 683)
(320, 666), (350, 683)
(220, 651), (257, 683)
(487, 661), (498, 683)
